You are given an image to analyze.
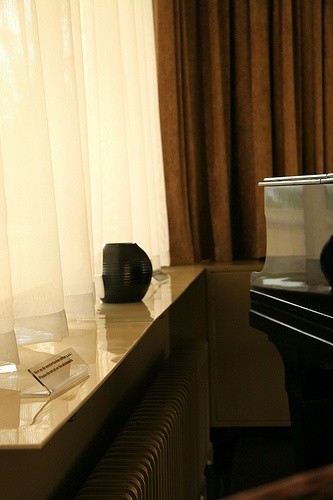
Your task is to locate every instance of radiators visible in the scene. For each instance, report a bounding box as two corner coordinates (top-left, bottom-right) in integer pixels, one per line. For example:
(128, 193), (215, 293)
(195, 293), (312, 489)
(75, 334), (212, 500)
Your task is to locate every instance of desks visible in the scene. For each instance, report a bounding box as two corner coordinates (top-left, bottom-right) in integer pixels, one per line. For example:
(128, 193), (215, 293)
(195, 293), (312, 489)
(0, 259), (212, 500)
